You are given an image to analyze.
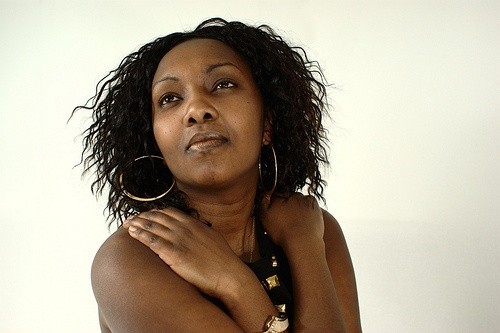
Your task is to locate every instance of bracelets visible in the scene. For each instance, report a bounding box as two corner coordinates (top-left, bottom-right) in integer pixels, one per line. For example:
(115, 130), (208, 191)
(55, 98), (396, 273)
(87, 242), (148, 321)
(259, 311), (290, 332)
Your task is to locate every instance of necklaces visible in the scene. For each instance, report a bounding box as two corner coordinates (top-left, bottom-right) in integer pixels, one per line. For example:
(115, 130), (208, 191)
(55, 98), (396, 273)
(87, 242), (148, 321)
(249, 216), (256, 262)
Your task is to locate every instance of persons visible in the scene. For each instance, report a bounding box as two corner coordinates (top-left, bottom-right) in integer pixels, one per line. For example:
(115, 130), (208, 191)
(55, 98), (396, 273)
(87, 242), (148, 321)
(66, 17), (362, 333)
